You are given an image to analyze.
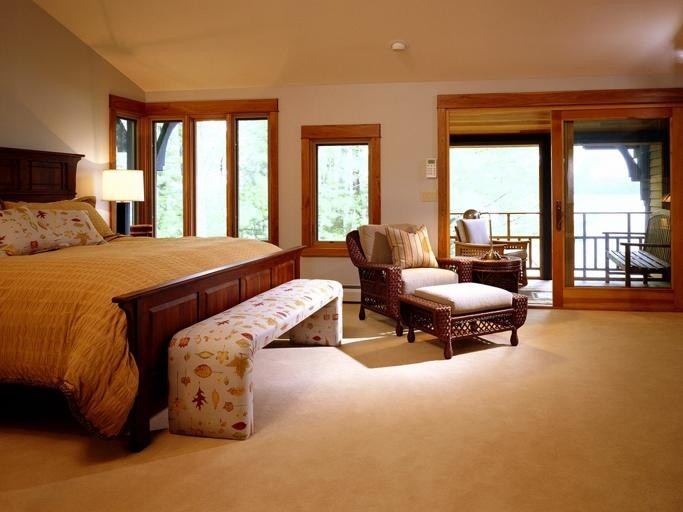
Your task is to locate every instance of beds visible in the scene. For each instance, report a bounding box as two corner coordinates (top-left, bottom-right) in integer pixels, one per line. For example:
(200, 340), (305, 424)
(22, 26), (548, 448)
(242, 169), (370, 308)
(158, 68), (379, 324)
(0, 145), (307, 454)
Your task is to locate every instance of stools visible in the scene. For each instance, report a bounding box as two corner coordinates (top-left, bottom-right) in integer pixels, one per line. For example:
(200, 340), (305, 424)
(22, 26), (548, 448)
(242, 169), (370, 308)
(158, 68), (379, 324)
(398, 281), (528, 360)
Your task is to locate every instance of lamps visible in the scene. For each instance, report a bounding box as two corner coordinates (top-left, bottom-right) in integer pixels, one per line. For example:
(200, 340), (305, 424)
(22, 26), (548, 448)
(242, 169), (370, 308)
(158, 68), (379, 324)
(99, 169), (144, 236)
(463, 210), (502, 261)
(661, 193), (670, 204)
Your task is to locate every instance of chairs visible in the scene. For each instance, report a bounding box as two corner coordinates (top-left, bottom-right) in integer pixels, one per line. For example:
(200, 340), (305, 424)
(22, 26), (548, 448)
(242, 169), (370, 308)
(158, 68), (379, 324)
(453, 218), (530, 285)
(345, 224), (473, 337)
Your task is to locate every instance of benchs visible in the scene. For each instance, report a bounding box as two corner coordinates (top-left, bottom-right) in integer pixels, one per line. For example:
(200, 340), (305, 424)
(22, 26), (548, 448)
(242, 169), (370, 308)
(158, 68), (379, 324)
(165, 278), (344, 441)
(601, 209), (670, 288)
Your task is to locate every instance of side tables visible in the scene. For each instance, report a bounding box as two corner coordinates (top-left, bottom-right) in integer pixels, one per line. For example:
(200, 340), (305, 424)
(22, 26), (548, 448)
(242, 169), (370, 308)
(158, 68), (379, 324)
(438, 255), (522, 293)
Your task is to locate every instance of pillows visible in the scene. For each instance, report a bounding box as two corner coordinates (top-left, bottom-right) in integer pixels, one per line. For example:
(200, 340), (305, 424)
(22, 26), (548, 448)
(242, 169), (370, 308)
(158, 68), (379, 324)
(0, 196), (118, 256)
(384, 224), (438, 269)
